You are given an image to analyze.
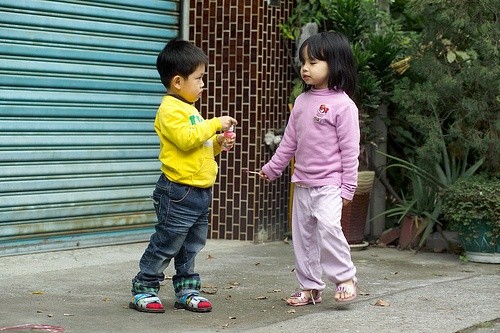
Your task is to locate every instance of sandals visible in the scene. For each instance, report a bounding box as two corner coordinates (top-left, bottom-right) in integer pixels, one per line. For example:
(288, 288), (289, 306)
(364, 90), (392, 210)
(174, 292), (213, 312)
(334, 277), (358, 301)
(129, 292), (167, 313)
(287, 288), (322, 306)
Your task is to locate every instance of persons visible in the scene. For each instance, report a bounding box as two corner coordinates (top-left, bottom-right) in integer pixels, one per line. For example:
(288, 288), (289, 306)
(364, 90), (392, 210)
(259, 33), (359, 306)
(130, 39), (237, 313)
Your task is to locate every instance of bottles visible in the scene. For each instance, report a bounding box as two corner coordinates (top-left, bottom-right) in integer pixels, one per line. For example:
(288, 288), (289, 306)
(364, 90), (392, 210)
(222, 125), (234, 151)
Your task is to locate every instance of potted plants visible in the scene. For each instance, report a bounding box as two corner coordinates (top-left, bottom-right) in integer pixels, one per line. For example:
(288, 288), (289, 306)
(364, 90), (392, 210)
(435, 176), (500, 253)
(339, 38), (376, 244)
(365, 156), (443, 251)
